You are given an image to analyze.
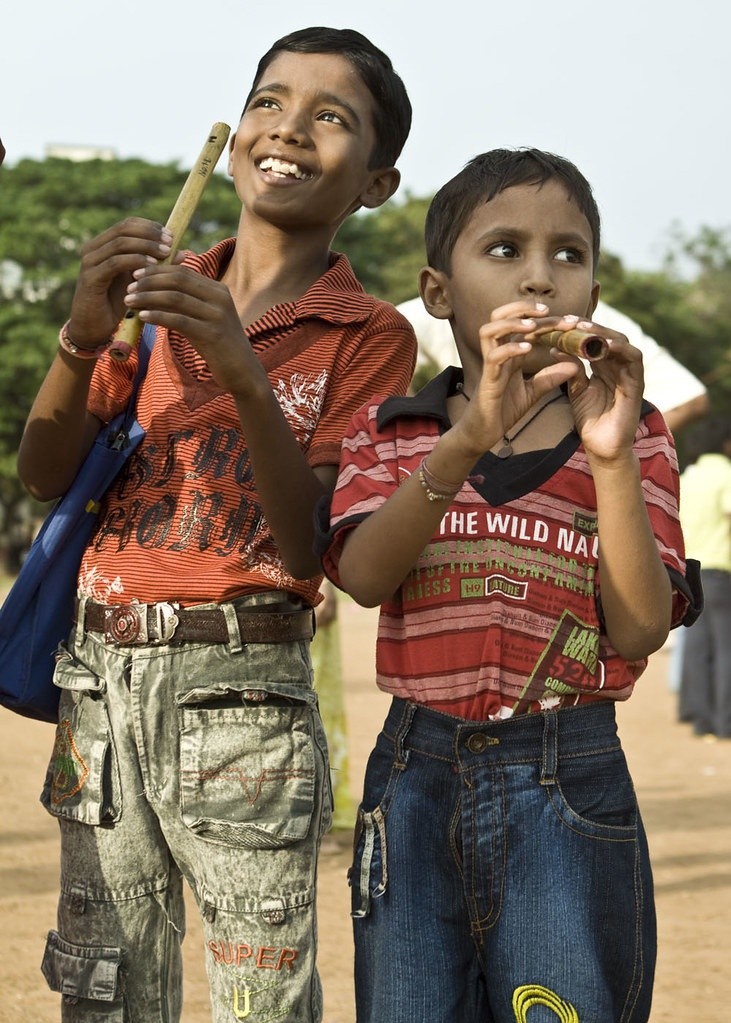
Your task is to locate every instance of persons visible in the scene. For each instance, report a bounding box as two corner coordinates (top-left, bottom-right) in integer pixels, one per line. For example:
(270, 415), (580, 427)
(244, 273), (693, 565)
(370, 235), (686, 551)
(392, 294), (709, 432)
(20, 27), (416, 1023)
(679, 421), (731, 742)
(323, 148), (685, 1023)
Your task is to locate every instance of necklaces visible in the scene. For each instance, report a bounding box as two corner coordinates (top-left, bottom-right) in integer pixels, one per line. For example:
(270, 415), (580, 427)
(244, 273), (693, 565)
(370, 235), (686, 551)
(456, 383), (568, 458)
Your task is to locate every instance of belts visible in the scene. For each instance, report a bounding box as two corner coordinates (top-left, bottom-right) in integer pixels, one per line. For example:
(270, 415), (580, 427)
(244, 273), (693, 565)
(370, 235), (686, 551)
(73, 599), (313, 646)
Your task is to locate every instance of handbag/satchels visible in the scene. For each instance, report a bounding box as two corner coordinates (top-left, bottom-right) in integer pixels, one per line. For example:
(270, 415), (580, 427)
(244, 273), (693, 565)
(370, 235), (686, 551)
(0, 322), (158, 724)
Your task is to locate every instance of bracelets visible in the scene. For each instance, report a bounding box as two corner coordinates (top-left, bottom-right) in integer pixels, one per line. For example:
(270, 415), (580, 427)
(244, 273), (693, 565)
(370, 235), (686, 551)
(60, 319), (114, 361)
(419, 456), (487, 502)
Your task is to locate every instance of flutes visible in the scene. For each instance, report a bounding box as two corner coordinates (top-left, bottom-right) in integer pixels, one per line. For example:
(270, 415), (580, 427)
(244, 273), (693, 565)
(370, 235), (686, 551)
(539, 329), (610, 363)
(108, 122), (231, 362)
